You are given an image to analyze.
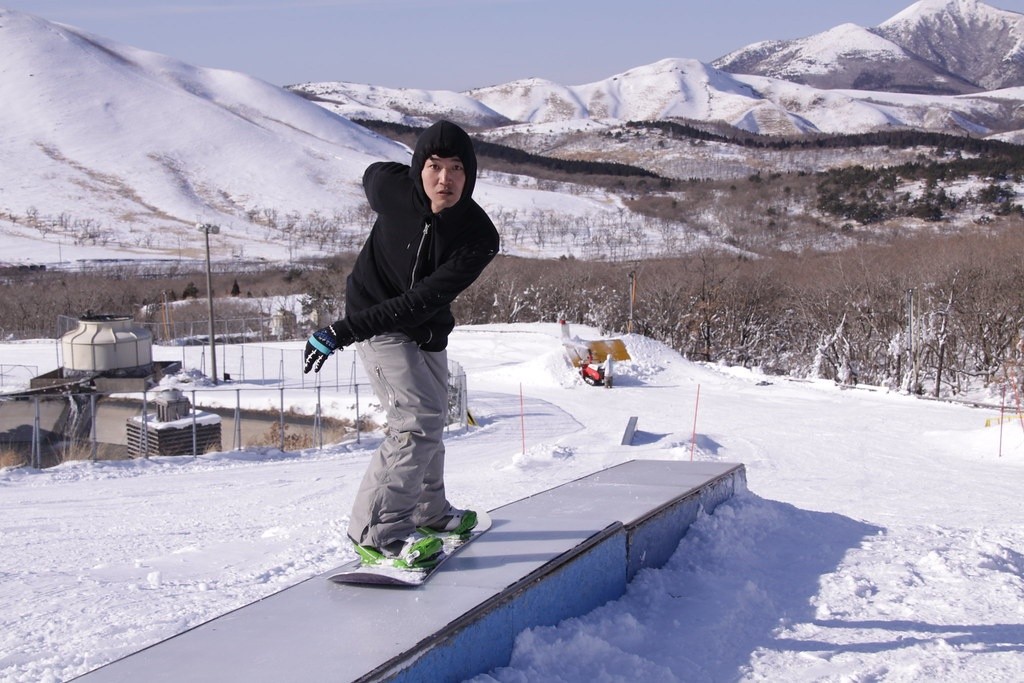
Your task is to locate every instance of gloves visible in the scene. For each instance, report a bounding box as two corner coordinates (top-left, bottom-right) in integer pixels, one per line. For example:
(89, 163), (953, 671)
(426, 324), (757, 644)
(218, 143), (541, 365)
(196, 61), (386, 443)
(304, 321), (355, 374)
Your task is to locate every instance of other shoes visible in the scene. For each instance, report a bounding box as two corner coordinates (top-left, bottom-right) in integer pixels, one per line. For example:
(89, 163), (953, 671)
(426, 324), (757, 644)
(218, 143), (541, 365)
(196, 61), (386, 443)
(347, 530), (445, 565)
(418, 505), (479, 532)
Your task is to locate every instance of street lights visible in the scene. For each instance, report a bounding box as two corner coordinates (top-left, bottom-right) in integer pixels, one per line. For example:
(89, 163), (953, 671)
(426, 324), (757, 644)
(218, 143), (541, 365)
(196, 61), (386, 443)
(195, 223), (220, 384)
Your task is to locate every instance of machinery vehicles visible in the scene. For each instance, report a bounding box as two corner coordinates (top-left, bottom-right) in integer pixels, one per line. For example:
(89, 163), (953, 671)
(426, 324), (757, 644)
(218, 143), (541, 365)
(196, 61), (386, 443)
(578, 349), (605, 386)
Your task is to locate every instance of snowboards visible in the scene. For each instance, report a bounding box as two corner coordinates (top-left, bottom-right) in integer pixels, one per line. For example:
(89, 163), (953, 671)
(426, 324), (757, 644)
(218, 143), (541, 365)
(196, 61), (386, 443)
(325, 507), (493, 586)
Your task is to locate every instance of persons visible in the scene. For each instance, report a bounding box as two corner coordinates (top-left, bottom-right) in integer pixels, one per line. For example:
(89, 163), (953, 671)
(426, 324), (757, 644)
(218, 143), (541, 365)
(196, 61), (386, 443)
(304, 120), (500, 573)
(601, 354), (616, 388)
